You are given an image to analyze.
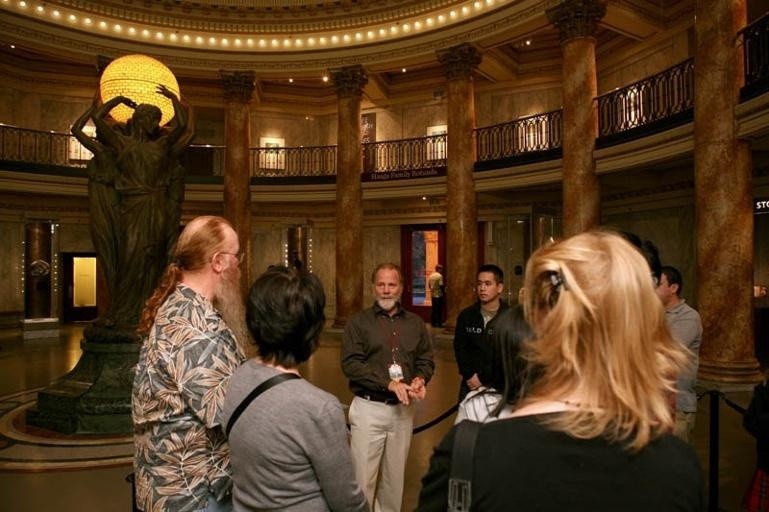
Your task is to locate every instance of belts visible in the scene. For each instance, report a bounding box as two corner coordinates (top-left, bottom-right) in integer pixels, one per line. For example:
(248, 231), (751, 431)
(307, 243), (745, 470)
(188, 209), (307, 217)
(359, 391), (400, 406)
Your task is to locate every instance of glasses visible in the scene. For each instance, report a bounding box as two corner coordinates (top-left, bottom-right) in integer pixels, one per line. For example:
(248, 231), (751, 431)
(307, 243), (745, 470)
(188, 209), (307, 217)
(222, 250), (245, 264)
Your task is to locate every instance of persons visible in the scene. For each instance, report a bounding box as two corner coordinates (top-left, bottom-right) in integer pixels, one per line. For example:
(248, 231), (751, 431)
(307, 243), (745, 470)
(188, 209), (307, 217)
(70, 83), (195, 329)
